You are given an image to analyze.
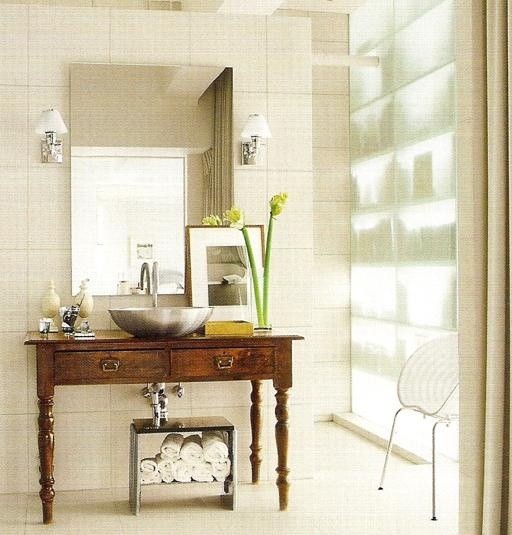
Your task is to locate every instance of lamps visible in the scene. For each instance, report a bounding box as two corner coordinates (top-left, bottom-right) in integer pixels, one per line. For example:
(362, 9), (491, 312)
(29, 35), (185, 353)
(34, 106), (69, 166)
(238, 111), (271, 170)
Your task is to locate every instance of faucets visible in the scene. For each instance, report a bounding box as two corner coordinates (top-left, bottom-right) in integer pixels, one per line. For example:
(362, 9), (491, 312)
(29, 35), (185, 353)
(150, 258), (159, 306)
(138, 261), (151, 294)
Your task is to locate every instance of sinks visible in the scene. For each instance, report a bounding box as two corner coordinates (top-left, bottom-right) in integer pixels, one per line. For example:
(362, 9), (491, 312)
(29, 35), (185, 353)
(108, 307), (213, 335)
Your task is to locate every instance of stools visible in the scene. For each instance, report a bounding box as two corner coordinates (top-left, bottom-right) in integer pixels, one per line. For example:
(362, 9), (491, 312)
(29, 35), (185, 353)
(125, 416), (241, 516)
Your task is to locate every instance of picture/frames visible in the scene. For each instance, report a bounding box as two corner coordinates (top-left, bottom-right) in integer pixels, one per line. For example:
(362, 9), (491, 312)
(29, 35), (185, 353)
(185, 223), (268, 331)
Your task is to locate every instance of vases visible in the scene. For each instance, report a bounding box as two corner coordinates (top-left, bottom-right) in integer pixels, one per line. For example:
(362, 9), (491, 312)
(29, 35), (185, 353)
(246, 261), (273, 329)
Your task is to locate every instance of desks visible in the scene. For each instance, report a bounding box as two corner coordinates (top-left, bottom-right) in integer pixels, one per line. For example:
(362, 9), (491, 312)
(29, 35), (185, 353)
(22, 328), (306, 524)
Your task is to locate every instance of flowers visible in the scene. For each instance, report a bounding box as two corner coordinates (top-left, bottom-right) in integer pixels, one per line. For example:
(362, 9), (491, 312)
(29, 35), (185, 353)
(225, 194), (290, 328)
(201, 209), (239, 228)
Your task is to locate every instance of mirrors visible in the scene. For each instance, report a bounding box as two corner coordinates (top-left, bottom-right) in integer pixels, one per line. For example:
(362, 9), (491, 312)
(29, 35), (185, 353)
(67, 61), (236, 329)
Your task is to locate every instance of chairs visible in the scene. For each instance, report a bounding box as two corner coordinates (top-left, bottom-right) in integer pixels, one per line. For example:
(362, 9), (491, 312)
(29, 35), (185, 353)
(378, 330), (460, 524)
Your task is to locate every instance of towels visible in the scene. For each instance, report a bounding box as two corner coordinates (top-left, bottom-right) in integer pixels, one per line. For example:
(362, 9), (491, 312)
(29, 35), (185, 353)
(140, 431), (231, 486)
